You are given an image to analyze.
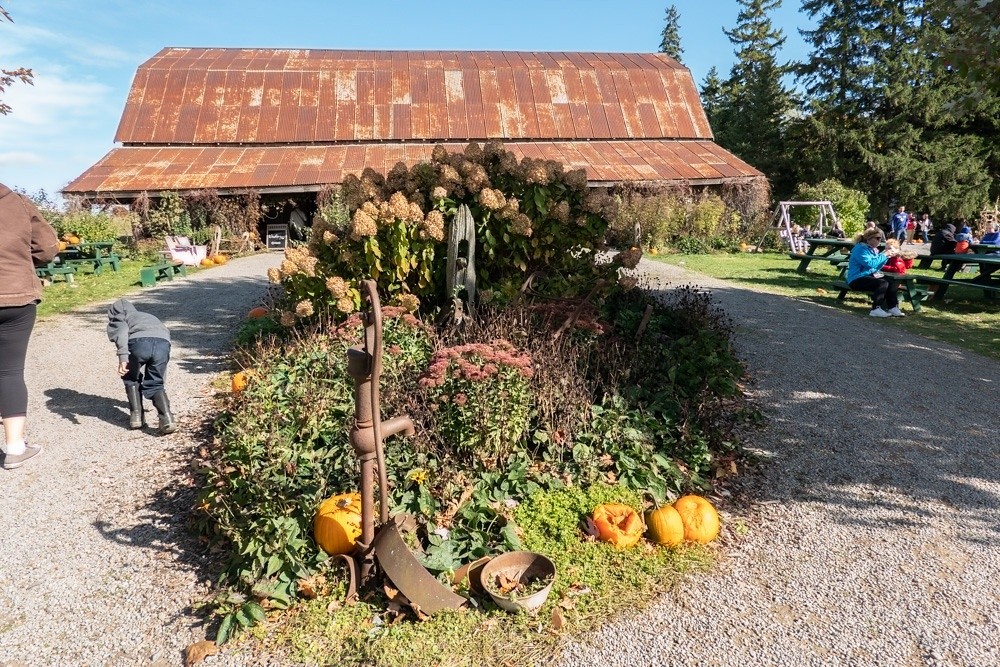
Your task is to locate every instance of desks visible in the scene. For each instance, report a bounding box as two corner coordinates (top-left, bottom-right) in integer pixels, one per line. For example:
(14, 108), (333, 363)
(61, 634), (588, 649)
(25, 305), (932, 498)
(66, 242), (114, 275)
(797, 238), (1000, 302)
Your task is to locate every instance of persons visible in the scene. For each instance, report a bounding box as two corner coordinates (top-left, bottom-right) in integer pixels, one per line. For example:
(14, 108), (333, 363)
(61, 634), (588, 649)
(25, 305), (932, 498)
(793, 205), (1000, 318)
(106, 297), (178, 436)
(0, 183), (60, 470)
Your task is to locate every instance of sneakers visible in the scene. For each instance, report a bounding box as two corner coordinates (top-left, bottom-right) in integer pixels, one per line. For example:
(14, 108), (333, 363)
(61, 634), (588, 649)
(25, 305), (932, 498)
(4, 444), (40, 469)
(868, 306), (892, 317)
(888, 304), (905, 316)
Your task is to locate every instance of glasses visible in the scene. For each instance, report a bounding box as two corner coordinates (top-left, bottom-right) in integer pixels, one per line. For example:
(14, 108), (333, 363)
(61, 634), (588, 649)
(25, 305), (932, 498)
(872, 237), (882, 241)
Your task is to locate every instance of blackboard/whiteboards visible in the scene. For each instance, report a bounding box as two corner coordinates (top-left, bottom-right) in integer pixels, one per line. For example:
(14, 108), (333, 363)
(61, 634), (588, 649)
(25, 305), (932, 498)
(267, 224), (291, 250)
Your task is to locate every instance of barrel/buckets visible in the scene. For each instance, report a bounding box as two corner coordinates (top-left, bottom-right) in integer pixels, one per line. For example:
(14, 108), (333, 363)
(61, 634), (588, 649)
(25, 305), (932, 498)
(480, 550), (555, 614)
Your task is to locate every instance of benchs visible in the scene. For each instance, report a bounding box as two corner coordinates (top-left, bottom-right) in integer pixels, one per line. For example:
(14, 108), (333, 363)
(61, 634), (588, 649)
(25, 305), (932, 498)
(788, 252), (1000, 313)
(34, 254), (121, 287)
(141, 235), (208, 286)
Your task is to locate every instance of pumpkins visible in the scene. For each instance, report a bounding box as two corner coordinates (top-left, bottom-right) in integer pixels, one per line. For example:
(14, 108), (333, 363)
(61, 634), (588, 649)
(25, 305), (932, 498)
(230, 367), (256, 392)
(593, 495), (719, 547)
(739, 243), (746, 248)
(248, 307), (266, 316)
(201, 259), (211, 265)
(650, 248), (657, 254)
(58, 236), (79, 250)
(912, 238), (923, 243)
(214, 255), (226, 263)
(314, 491), (364, 556)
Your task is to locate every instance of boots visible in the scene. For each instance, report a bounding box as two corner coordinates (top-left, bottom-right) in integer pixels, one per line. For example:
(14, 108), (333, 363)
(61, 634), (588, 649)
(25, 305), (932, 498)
(152, 390), (176, 432)
(124, 382), (146, 428)
